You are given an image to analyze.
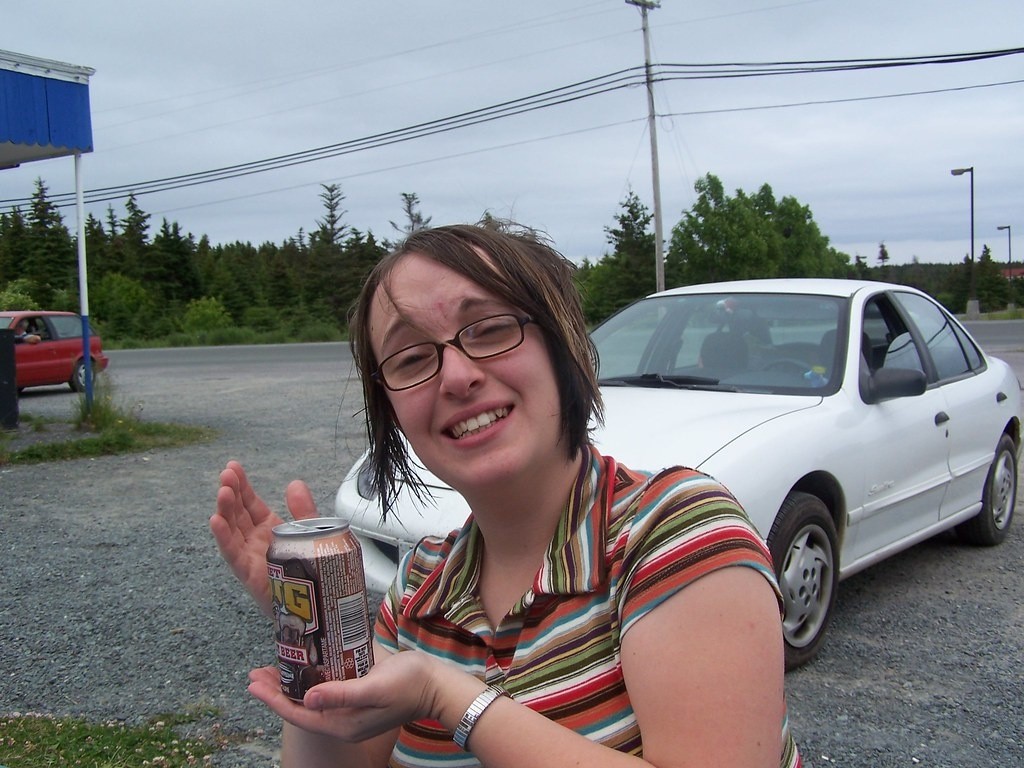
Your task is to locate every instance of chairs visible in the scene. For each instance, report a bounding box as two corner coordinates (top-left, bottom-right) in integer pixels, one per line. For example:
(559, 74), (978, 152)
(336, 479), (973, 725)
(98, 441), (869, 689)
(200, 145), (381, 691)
(682, 329), (883, 392)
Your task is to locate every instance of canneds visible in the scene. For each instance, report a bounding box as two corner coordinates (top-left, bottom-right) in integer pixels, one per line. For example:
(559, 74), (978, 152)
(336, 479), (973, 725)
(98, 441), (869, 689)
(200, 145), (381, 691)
(266, 518), (375, 704)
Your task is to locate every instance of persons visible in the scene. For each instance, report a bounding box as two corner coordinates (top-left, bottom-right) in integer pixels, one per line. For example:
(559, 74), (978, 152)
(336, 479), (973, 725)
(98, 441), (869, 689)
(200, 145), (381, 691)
(13, 320), (41, 343)
(210, 223), (804, 768)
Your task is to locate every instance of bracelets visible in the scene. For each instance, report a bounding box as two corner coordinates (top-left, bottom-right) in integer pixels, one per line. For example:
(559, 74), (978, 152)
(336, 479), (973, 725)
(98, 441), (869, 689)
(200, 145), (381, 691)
(452, 683), (514, 754)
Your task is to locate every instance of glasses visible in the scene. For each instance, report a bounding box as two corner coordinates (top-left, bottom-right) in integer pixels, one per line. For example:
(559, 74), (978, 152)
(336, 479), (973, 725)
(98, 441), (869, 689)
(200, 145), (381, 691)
(369, 314), (538, 392)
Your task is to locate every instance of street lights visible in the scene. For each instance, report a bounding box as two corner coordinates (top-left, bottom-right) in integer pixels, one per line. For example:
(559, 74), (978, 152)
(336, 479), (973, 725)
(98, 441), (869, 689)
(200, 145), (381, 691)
(997, 226), (1011, 301)
(951, 165), (977, 298)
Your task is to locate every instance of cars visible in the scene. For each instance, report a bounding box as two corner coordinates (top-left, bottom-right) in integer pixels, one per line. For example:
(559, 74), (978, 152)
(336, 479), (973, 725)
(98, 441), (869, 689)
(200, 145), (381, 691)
(336, 275), (1024, 669)
(0, 309), (109, 392)
